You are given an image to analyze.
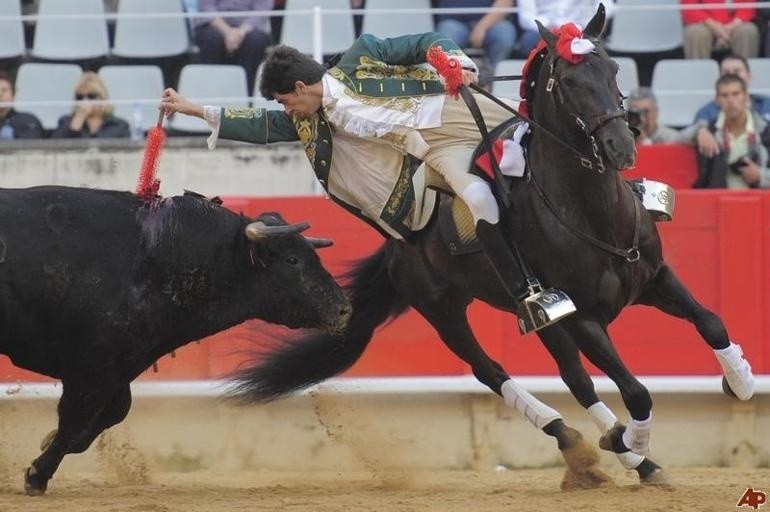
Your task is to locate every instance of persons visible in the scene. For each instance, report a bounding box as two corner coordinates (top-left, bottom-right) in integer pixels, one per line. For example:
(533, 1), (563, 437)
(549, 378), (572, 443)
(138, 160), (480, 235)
(680, 1), (761, 59)
(625, 87), (679, 147)
(184, 1), (276, 100)
(517, 0), (616, 58)
(50, 71), (132, 138)
(693, 76), (770, 188)
(435, 0), (519, 88)
(0, 71), (44, 139)
(155, 33), (579, 335)
(675, 55), (770, 159)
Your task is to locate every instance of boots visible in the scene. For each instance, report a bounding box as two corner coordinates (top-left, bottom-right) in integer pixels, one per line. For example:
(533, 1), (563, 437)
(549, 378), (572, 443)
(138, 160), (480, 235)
(474, 218), (532, 302)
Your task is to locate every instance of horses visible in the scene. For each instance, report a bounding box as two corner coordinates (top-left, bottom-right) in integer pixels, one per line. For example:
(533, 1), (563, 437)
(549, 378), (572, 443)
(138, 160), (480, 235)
(203, 2), (757, 493)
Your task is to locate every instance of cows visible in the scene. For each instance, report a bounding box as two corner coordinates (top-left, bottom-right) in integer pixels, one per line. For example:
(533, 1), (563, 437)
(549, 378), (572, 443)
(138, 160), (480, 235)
(0, 184), (354, 497)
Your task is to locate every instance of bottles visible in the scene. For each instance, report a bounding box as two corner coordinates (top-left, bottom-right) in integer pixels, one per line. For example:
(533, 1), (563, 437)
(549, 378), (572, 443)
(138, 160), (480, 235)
(129, 102), (143, 141)
